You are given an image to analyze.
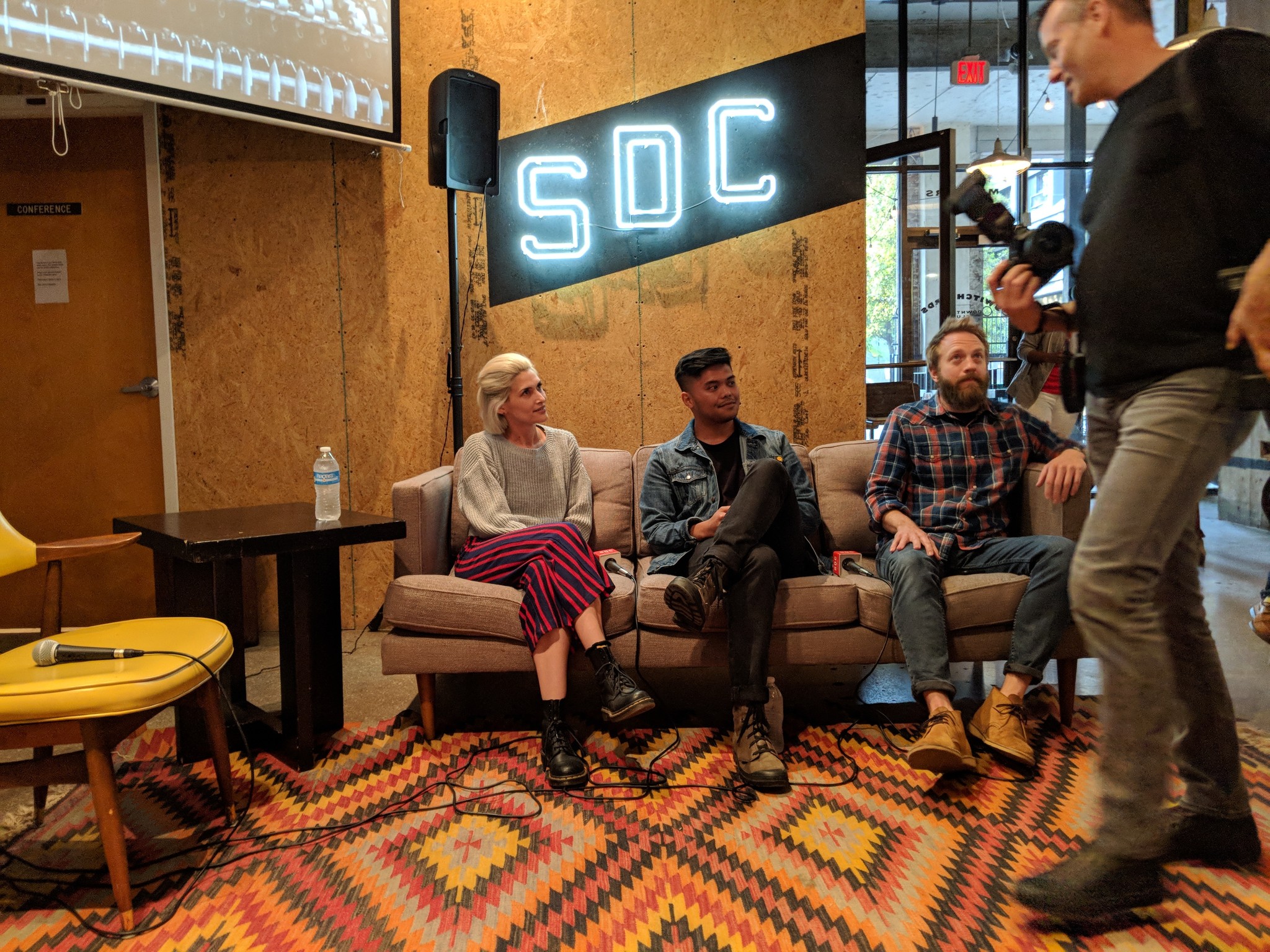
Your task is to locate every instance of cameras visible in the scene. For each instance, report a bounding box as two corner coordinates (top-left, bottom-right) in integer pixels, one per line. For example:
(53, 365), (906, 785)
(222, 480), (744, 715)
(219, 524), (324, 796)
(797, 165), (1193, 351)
(942, 168), (1076, 297)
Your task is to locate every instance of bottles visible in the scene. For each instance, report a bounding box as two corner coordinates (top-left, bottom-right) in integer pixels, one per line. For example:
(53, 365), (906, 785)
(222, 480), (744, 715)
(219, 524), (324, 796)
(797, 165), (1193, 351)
(314, 447), (342, 521)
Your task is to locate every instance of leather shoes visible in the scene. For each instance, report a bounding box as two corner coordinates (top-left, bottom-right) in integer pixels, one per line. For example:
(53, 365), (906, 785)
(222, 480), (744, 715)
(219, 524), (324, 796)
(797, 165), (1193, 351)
(1016, 803), (1262, 915)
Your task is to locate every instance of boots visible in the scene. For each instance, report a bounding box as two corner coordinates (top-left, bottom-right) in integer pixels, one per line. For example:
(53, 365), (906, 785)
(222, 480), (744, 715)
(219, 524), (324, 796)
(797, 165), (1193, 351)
(539, 699), (589, 787)
(591, 646), (656, 723)
(729, 698), (790, 788)
(907, 683), (1036, 771)
(663, 555), (728, 634)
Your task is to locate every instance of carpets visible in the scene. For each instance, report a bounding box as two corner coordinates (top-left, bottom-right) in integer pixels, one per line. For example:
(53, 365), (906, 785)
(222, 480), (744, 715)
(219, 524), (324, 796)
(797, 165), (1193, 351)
(2, 691), (1270, 952)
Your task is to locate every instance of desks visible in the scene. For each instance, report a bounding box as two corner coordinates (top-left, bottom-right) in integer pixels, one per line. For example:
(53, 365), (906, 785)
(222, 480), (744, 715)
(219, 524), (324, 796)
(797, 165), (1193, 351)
(112, 502), (408, 772)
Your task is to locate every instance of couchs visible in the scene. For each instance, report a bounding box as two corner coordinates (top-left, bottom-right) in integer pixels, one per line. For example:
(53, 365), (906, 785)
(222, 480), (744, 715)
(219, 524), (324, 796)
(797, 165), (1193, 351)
(378, 440), (1093, 745)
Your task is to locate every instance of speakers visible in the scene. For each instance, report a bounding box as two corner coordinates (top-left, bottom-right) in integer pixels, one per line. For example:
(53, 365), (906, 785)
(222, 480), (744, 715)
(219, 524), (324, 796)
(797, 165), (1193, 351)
(426, 68), (502, 197)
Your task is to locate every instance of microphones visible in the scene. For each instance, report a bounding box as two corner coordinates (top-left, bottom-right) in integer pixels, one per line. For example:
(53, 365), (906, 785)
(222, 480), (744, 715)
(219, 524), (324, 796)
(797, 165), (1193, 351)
(32, 639), (147, 667)
(832, 550), (875, 578)
(593, 548), (633, 580)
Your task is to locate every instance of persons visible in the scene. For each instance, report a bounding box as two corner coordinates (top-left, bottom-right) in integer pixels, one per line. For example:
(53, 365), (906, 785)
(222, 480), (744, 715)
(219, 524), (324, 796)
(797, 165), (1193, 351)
(860, 315), (1097, 782)
(985, 2), (1270, 923)
(1005, 296), (1085, 440)
(454, 352), (655, 788)
(635, 345), (838, 793)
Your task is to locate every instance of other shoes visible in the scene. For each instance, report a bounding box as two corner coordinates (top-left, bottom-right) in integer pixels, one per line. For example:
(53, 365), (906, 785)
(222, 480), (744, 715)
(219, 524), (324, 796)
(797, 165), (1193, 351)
(1249, 596), (1270, 645)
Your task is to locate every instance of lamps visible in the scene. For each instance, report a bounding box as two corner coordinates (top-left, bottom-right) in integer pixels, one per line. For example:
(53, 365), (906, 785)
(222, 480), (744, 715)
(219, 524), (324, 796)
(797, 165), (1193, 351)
(1164, 5), (1257, 51)
(1044, 96), (1053, 112)
(967, 138), (1032, 176)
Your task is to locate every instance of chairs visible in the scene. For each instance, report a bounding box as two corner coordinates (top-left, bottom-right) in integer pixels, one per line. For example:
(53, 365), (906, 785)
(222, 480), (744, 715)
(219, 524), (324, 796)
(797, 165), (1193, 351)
(2, 507), (240, 936)
